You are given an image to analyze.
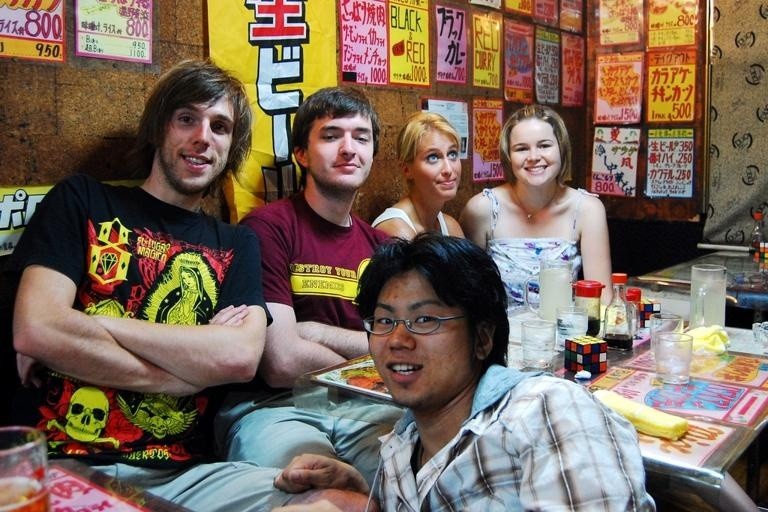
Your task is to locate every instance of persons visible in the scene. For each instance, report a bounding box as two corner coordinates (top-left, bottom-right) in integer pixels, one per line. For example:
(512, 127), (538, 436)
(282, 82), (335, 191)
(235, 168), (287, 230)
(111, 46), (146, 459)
(237, 87), (411, 512)
(371, 110), (465, 243)
(270, 231), (659, 512)
(459, 108), (613, 317)
(4, 57), (374, 512)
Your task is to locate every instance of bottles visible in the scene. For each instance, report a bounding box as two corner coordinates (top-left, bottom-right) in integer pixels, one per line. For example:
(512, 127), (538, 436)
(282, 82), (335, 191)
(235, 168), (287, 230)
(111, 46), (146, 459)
(749, 213), (764, 256)
(572, 279), (605, 337)
(603, 272), (635, 349)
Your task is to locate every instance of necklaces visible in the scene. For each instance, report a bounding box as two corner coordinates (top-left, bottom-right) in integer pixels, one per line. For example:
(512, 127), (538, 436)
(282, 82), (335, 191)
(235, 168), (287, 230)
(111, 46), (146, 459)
(407, 194), (438, 235)
(508, 180), (559, 221)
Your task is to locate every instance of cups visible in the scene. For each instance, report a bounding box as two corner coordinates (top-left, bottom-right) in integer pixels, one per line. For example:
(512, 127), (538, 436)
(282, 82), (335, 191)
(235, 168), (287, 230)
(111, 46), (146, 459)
(574, 279), (602, 338)
(521, 320), (556, 369)
(0, 427), (51, 512)
(555, 305), (588, 352)
(689, 263), (727, 329)
(654, 332), (693, 385)
(523, 260), (573, 322)
(649, 313), (684, 357)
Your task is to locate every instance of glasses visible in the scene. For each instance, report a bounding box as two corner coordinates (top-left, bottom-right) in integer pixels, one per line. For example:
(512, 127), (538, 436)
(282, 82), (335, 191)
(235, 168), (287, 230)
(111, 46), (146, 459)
(362, 312), (466, 335)
